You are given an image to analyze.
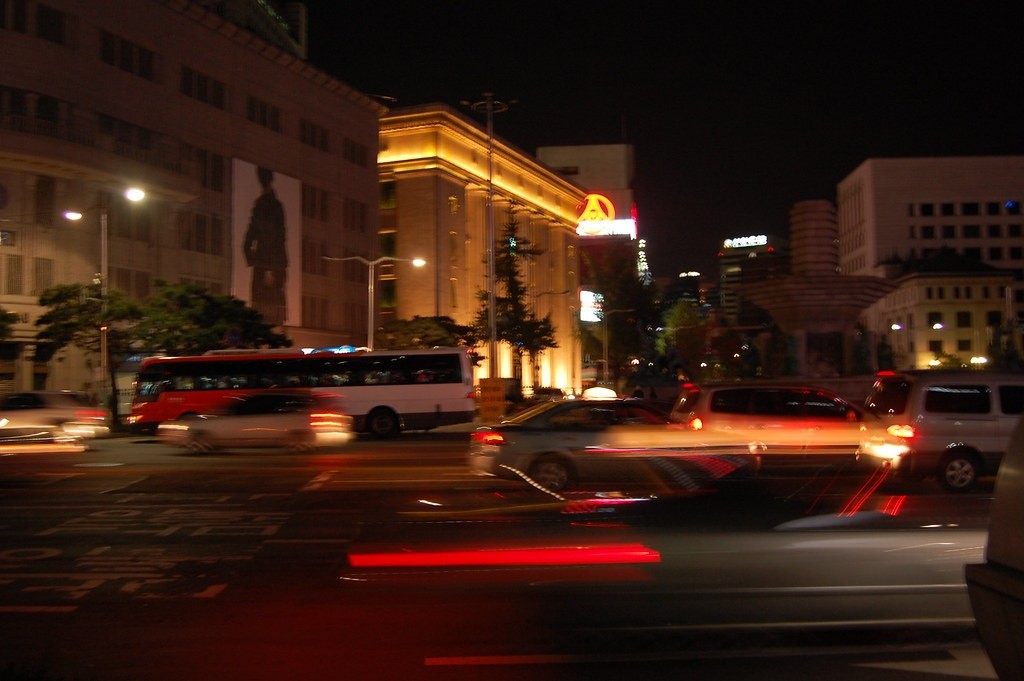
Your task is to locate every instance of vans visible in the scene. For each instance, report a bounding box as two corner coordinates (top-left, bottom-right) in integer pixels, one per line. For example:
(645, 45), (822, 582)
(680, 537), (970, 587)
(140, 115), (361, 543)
(857, 368), (1024, 496)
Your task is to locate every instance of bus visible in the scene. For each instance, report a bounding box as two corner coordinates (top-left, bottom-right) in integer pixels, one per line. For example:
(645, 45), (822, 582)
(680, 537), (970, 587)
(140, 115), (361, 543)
(127, 347), (479, 447)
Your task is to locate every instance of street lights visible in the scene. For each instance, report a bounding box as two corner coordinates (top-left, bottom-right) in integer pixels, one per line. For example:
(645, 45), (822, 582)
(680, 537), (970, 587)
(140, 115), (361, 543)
(61, 179), (145, 397)
(320, 256), (426, 351)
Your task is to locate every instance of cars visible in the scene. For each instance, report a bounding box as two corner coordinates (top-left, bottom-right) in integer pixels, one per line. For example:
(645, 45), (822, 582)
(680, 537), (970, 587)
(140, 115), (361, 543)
(666, 379), (879, 480)
(0, 390), (113, 443)
(469, 393), (767, 502)
